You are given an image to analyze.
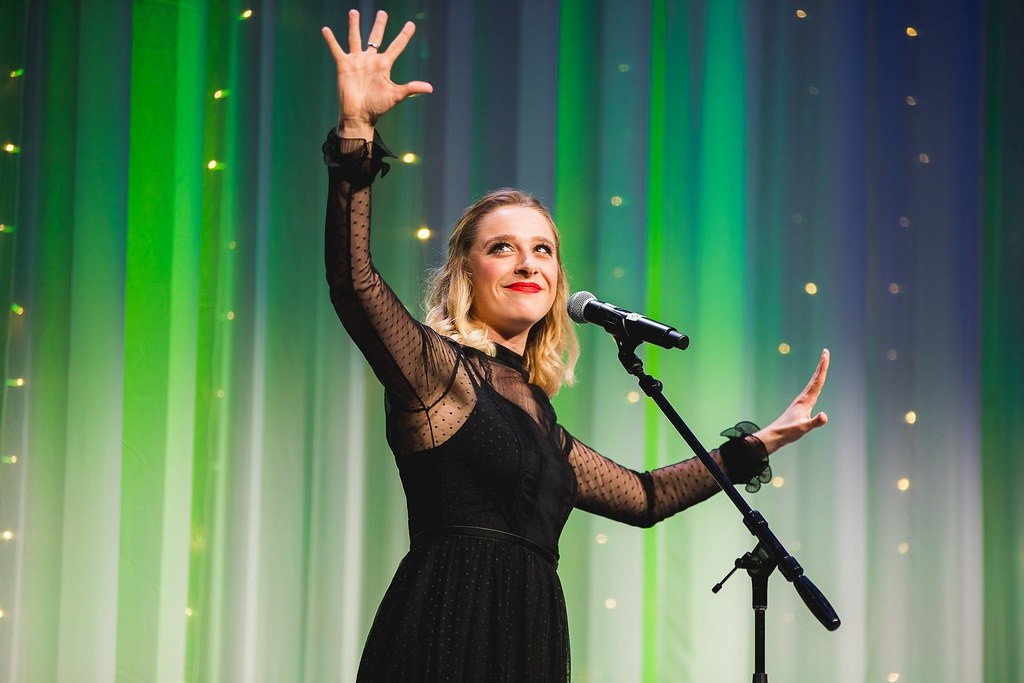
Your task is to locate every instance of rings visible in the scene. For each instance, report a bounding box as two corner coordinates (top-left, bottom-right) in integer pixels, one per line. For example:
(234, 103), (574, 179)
(366, 42), (382, 53)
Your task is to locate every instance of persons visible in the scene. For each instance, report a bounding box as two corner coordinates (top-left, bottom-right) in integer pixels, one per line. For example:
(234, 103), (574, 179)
(320, 8), (831, 683)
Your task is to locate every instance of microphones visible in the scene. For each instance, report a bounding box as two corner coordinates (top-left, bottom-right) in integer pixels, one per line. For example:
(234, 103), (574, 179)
(568, 290), (690, 350)
